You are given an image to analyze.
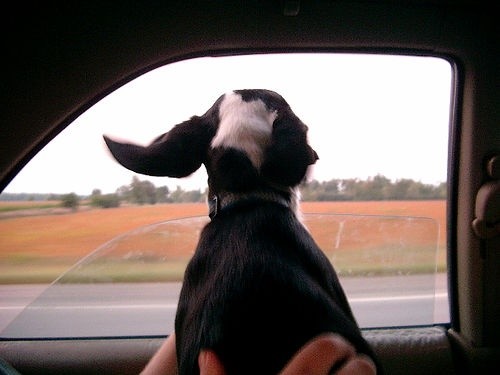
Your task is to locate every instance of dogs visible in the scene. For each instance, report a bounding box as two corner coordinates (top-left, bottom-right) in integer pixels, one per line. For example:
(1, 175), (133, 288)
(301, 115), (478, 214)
(101, 87), (384, 375)
